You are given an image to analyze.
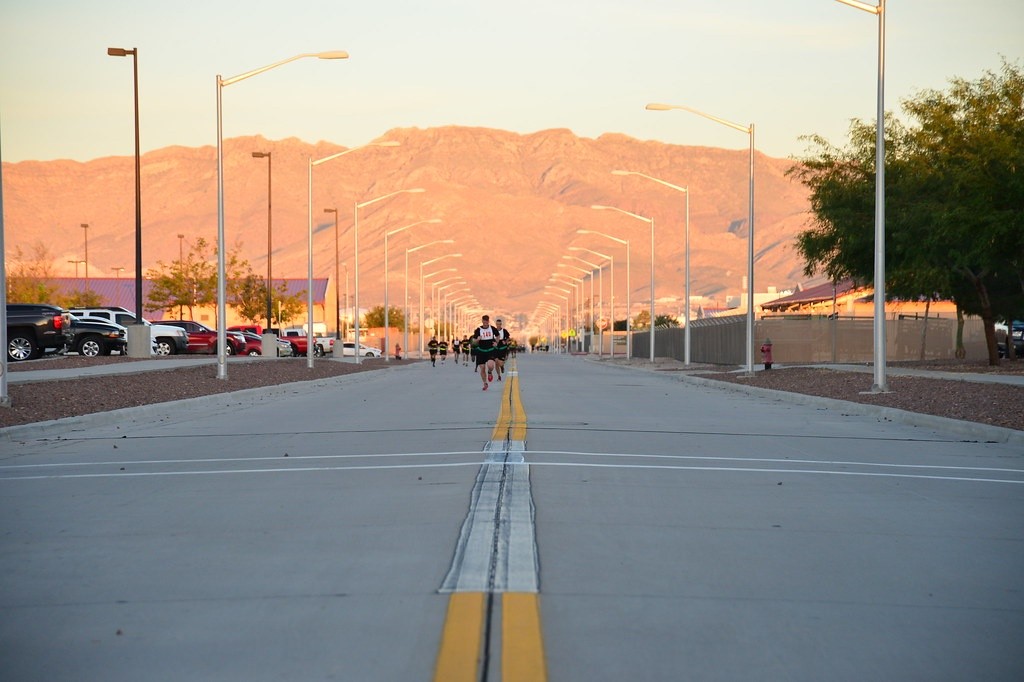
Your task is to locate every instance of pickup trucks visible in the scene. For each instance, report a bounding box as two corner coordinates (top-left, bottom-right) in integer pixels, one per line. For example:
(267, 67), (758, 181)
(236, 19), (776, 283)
(282, 329), (335, 357)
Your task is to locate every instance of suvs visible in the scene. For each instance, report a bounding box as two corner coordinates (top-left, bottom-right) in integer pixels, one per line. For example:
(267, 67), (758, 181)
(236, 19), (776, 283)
(7, 303), (74, 360)
(150, 322), (247, 356)
(68, 306), (189, 356)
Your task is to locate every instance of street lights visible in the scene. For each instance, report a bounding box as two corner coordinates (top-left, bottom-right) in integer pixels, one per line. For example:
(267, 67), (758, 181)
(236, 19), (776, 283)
(404, 239), (495, 360)
(592, 205), (656, 363)
(646, 100), (756, 379)
(521, 228), (631, 361)
(250, 150), (273, 334)
(384, 218), (443, 362)
(216, 50), (345, 381)
(108, 47), (146, 326)
(322, 208), (341, 339)
(354, 187), (425, 364)
(613, 171), (693, 366)
(304, 139), (404, 368)
(178, 234), (184, 270)
(111, 267), (124, 277)
(68, 223), (89, 293)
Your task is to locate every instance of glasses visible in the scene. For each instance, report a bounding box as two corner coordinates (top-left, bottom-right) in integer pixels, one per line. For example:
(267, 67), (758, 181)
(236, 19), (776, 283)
(482, 318), (489, 321)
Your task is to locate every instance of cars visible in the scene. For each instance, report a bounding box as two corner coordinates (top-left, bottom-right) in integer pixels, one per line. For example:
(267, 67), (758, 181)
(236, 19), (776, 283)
(227, 327), (293, 358)
(343, 342), (382, 358)
(993, 318), (1024, 359)
(66, 316), (128, 358)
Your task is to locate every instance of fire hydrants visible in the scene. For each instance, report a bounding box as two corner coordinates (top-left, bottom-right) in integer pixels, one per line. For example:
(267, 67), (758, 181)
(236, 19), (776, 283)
(760, 337), (774, 370)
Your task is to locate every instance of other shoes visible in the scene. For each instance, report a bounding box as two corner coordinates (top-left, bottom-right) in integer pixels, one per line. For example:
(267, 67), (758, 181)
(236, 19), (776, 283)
(466, 364), (468, 366)
(498, 375), (501, 381)
(432, 363), (435, 367)
(501, 366), (504, 373)
(475, 368), (477, 372)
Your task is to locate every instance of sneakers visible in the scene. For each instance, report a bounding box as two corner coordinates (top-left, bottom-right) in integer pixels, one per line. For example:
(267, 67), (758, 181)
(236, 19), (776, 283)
(483, 383), (488, 391)
(488, 370), (493, 382)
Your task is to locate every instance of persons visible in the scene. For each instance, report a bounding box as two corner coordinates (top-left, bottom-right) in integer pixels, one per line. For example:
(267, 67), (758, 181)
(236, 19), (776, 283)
(461, 335), (469, 366)
(468, 330), (478, 373)
(505, 337), (550, 360)
(472, 315), (499, 391)
(451, 337), (461, 364)
(438, 338), (448, 364)
(493, 319), (510, 381)
(427, 336), (438, 367)
